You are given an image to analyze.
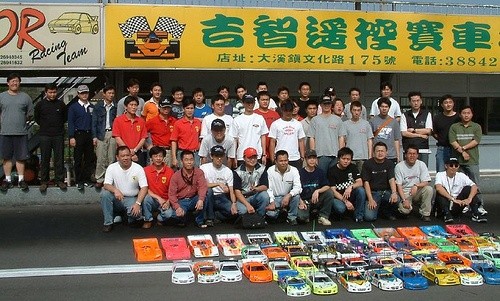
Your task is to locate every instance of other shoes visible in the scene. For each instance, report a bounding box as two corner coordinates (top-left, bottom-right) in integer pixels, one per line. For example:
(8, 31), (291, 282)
(77, 179), (90, 189)
(95, 182), (104, 188)
(57, 180), (67, 190)
(39, 180), (48, 192)
(17, 181), (29, 192)
(0, 180), (13, 191)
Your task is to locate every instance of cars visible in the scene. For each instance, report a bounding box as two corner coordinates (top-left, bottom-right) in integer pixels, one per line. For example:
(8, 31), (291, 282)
(365, 265), (404, 291)
(392, 266), (427, 290)
(268, 261), (297, 282)
(172, 260), (195, 285)
(261, 244), (290, 263)
(329, 233), (500, 274)
(219, 260), (243, 282)
(336, 268), (372, 293)
(241, 245), (269, 263)
(317, 257), (345, 278)
(291, 256), (320, 279)
(304, 240), (341, 261)
(194, 262), (221, 284)
(306, 272), (338, 295)
(277, 271), (312, 296)
(283, 244), (309, 257)
(471, 263), (500, 285)
(421, 264), (461, 286)
(446, 264), (484, 286)
(242, 262), (272, 282)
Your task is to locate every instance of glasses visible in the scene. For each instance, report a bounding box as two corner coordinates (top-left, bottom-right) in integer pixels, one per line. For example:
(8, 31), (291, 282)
(446, 163), (458, 168)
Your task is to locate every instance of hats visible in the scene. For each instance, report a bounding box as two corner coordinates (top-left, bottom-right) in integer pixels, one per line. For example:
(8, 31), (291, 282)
(243, 147), (257, 158)
(159, 98), (173, 108)
(211, 145), (225, 155)
(445, 156), (459, 164)
(242, 93), (255, 104)
(211, 119), (225, 131)
(77, 85), (89, 93)
(320, 94), (333, 104)
(324, 87), (337, 96)
(304, 150), (318, 159)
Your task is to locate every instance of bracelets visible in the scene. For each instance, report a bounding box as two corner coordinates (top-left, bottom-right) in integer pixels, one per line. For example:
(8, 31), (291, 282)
(402, 198), (407, 202)
(135, 201), (141, 206)
(462, 145), (466, 150)
(412, 128), (416, 133)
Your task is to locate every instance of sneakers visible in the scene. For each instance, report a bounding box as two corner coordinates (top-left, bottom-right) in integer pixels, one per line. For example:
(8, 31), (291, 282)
(100, 205), (488, 228)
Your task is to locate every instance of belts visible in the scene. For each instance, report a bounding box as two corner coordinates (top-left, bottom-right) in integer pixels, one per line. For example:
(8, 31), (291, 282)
(161, 146), (172, 150)
(180, 148), (200, 153)
(105, 129), (112, 132)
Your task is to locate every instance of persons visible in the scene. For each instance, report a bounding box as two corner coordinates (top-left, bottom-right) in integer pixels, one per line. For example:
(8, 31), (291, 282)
(292, 82), (319, 118)
(370, 83), (401, 121)
(142, 82), (162, 145)
(35, 83), (67, 190)
(218, 84), (233, 115)
(0, 73), (35, 191)
(193, 88), (211, 117)
(233, 85), (247, 113)
(68, 85), (94, 190)
(317, 86), (344, 116)
(170, 86), (184, 118)
(117, 79), (144, 115)
(342, 88), (366, 120)
(91, 84), (117, 189)
(253, 81), (289, 111)
(102, 91), (487, 231)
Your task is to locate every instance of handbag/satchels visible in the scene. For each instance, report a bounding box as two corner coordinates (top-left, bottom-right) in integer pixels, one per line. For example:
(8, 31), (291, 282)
(237, 213), (265, 229)
